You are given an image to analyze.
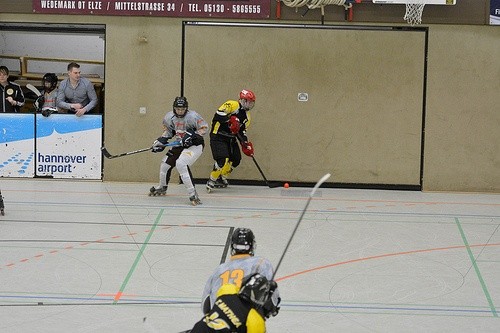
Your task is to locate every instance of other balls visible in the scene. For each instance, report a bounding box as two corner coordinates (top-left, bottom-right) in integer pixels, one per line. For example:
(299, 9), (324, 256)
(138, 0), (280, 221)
(284, 183), (288, 187)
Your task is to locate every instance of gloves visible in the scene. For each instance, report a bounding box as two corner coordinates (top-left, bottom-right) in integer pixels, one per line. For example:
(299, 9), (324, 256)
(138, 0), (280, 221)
(43, 107), (58, 117)
(227, 115), (240, 134)
(180, 129), (198, 148)
(151, 137), (168, 153)
(33, 94), (45, 109)
(241, 140), (254, 157)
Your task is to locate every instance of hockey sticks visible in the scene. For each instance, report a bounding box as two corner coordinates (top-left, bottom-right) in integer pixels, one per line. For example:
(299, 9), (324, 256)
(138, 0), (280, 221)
(100, 141), (181, 159)
(237, 132), (282, 187)
(270, 173), (332, 284)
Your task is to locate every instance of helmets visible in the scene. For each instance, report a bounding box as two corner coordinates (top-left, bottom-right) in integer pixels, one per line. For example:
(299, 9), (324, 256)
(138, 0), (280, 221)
(230, 227), (255, 257)
(238, 273), (269, 306)
(240, 90), (255, 102)
(41, 73), (58, 93)
(172, 97), (189, 118)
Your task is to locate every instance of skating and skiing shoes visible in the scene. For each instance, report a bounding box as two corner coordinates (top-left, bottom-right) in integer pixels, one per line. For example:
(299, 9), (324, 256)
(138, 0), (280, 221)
(148, 185), (167, 196)
(215, 176), (228, 188)
(205, 176), (215, 193)
(189, 191), (200, 206)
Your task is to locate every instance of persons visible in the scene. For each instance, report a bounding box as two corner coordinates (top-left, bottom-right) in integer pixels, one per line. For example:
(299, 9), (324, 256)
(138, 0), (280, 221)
(56, 62), (97, 116)
(149, 96), (208, 205)
(34, 73), (60, 115)
(201, 227), (273, 318)
(205, 89), (256, 192)
(182, 272), (280, 333)
(0, 65), (25, 113)
